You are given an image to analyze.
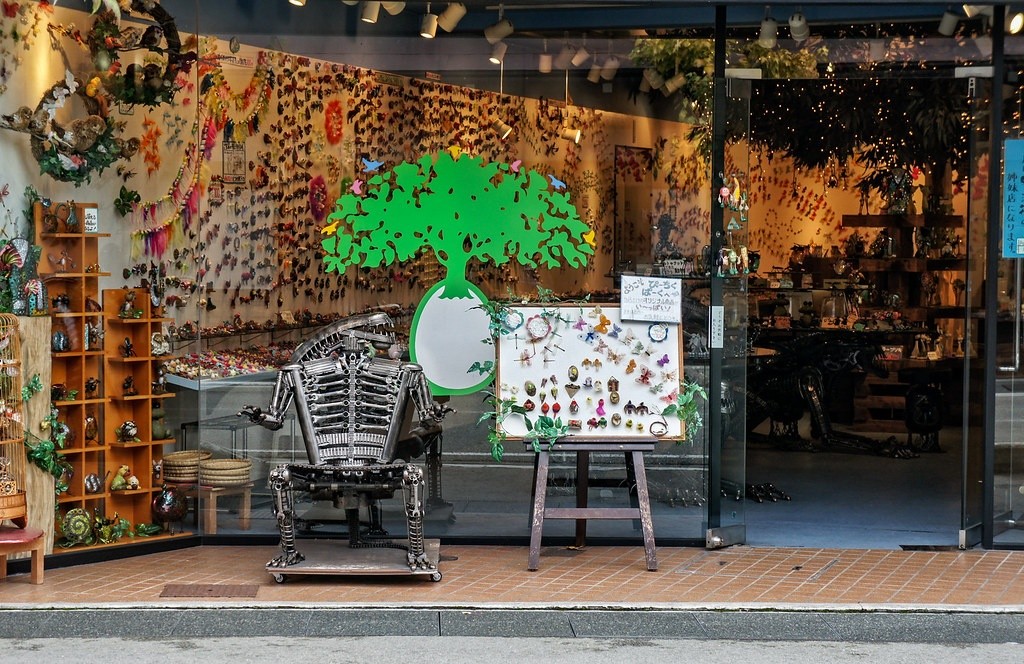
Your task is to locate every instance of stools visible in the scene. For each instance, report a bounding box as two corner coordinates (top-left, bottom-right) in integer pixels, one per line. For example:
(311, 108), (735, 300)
(0, 525), (45, 586)
(164, 478), (254, 534)
(521, 435), (660, 572)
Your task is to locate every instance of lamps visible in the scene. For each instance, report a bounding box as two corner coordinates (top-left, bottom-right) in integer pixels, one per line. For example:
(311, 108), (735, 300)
(937, 2), (1023, 37)
(285, 0), (811, 144)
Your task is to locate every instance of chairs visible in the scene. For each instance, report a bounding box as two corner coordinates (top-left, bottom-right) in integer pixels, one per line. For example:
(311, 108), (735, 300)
(232, 303), (457, 586)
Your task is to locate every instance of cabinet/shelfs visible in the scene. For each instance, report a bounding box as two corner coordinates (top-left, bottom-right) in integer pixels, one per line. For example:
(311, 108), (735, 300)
(746, 212), (984, 420)
(30, 200), (197, 563)
